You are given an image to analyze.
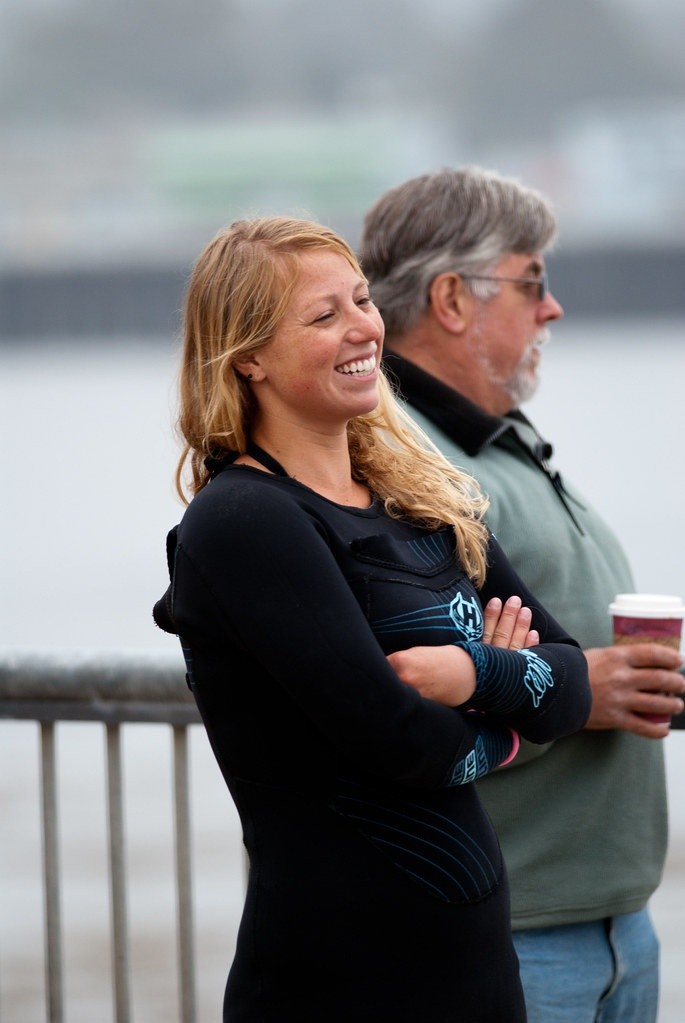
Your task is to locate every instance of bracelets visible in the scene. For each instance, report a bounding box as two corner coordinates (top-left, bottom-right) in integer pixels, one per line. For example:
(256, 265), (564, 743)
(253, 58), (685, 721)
(501, 728), (519, 767)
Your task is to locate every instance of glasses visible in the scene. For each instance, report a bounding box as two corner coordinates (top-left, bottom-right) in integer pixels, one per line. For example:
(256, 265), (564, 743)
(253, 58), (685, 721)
(456, 272), (549, 301)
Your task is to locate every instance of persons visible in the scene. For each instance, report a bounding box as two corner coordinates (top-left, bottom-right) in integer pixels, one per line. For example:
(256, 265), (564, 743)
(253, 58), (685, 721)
(153, 215), (593, 1023)
(353, 167), (685, 1023)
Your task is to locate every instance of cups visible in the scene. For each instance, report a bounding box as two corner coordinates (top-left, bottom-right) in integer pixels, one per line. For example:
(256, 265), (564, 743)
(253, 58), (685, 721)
(607, 592), (684, 731)
